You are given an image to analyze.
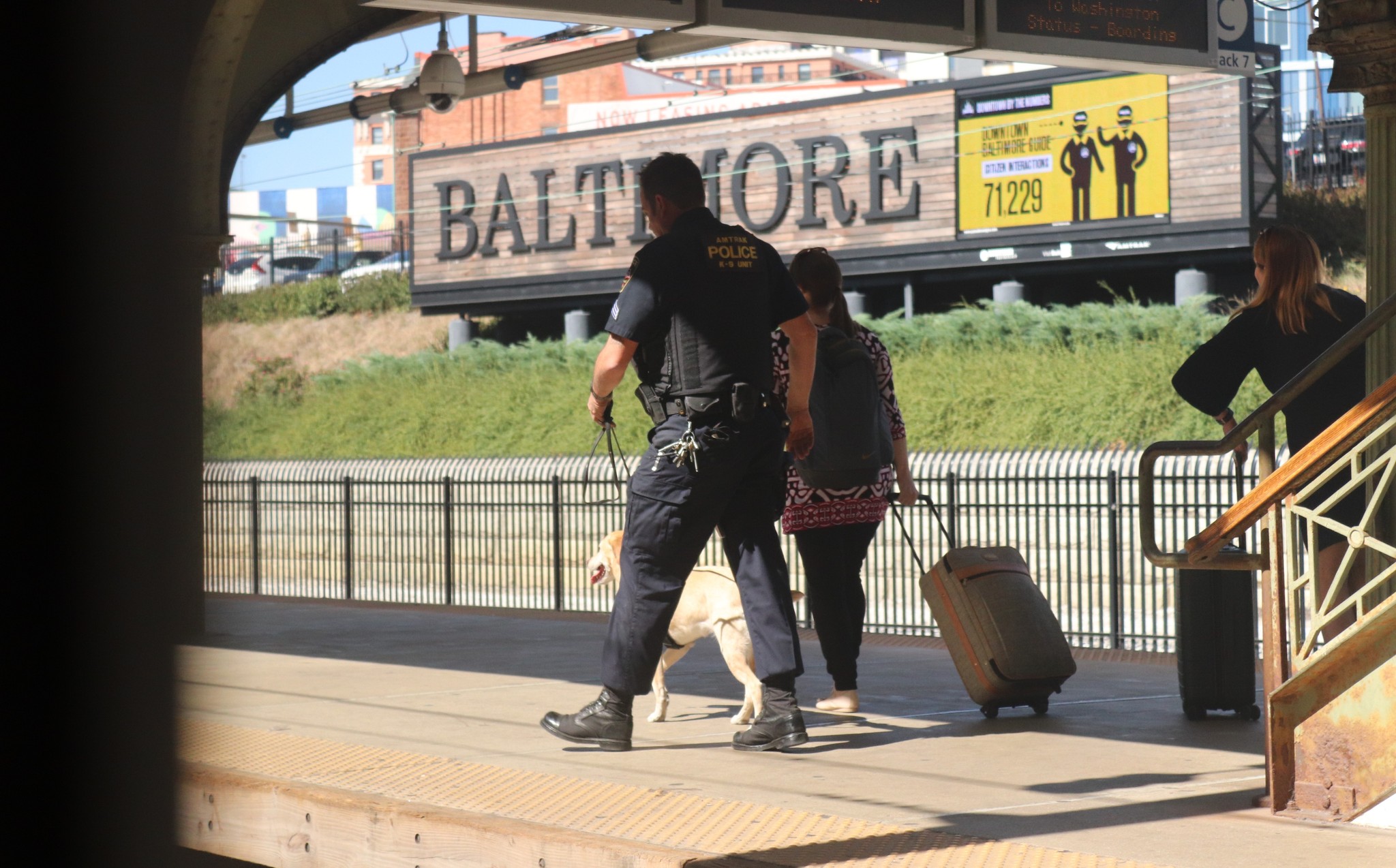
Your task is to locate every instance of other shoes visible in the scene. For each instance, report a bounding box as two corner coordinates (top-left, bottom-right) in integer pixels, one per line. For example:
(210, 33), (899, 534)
(816, 686), (858, 713)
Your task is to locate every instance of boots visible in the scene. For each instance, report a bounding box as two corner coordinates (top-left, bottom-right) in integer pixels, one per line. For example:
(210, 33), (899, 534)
(541, 687), (632, 751)
(732, 679), (808, 752)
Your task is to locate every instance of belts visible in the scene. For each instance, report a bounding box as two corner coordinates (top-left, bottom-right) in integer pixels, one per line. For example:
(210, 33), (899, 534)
(665, 399), (687, 416)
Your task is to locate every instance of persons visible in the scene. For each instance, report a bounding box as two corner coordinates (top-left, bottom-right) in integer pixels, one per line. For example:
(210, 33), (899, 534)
(770, 248), (920, 712)
(1174, 226), (1368, 645)
(540, 153), (818, 750)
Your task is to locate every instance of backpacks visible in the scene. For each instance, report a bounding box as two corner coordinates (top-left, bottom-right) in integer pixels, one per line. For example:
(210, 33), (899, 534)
(785, 320), (895, 488)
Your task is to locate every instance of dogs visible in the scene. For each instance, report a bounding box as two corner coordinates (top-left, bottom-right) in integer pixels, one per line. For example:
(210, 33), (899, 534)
(586, 531), (807, 727)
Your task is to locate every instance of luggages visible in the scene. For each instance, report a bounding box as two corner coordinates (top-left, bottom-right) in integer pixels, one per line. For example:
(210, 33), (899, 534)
(1175, 451), (1261, 723)
(887, 491), (1077, 719)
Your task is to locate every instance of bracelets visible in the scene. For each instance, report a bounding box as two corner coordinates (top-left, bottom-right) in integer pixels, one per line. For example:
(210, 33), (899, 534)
(1216, 408), (1234, 425)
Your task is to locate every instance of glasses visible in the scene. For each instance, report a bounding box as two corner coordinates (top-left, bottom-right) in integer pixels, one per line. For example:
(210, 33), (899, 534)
(795, 247), (829, 258)
(1260, 225), (1271, 260)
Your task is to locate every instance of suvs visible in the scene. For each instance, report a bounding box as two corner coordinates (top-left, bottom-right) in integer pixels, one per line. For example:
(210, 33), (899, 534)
(1287, 113), (1368, 186)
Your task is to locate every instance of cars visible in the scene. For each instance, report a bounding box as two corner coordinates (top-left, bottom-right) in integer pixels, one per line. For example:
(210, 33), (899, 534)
(223, 252), (341, 294)
(304, 250), (394, 284)
(342, 250), (408, 290)
